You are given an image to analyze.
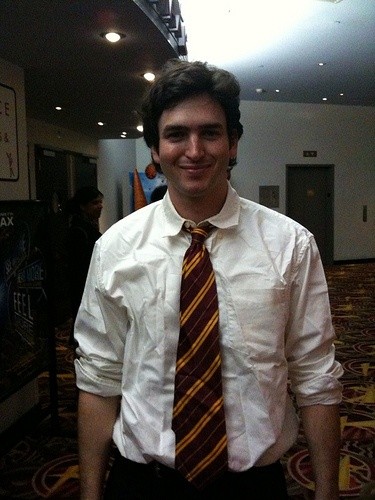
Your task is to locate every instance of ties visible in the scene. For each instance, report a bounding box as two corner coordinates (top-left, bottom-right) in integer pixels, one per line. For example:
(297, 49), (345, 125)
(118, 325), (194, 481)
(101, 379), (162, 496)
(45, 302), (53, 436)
(172, 222), (228, 495)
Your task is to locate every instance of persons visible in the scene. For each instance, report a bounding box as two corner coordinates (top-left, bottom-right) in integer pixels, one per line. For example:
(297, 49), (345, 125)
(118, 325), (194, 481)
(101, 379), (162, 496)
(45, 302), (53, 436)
(74, 58), (346, 499)
(71, 187), (103, 264)
